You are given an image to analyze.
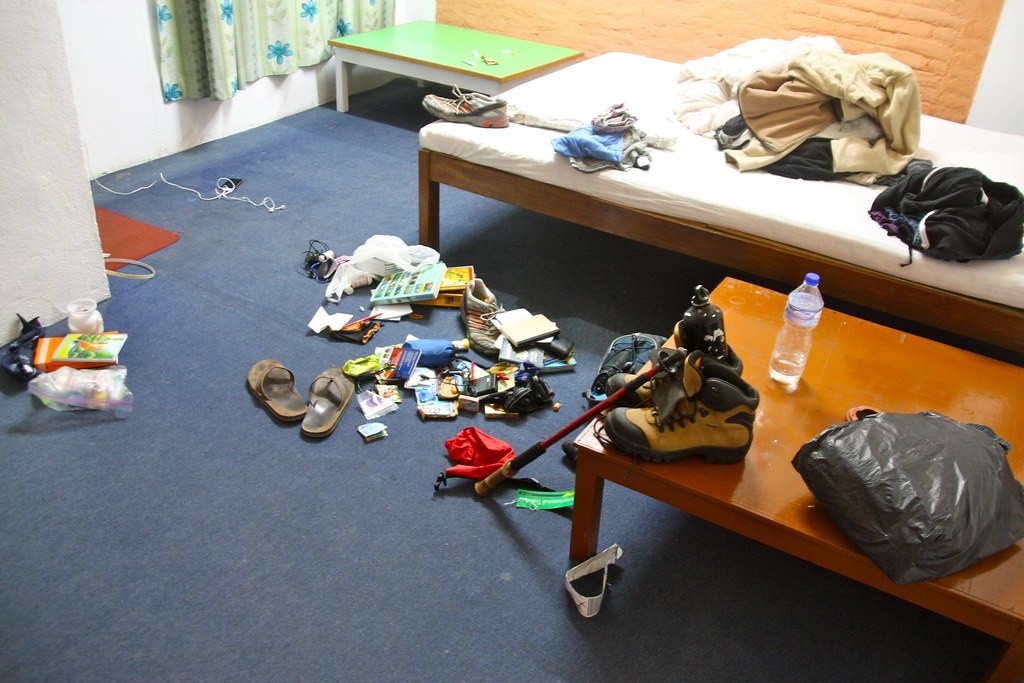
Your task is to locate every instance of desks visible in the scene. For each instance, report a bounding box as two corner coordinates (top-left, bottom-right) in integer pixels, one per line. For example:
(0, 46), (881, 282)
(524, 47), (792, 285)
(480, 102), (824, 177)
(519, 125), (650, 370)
(569, 276), (1024, 683)
(329, 21), (583, 112)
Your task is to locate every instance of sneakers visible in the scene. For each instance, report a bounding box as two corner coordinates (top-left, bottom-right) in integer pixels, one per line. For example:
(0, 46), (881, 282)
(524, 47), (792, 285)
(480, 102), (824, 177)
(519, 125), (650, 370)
(606, 342), (744, 408)
(421, 84), (509, 128)
(604, 351), (759, 464)
(460, 278), (504, 355)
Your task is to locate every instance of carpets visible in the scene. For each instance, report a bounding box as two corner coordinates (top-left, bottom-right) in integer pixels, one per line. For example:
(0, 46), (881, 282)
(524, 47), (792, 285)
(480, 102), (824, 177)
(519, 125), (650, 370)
(95, 206), (180, 270)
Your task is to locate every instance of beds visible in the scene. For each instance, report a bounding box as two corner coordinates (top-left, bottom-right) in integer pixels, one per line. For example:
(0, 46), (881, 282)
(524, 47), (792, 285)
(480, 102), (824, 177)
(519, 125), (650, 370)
(420, 52), (1024, 354)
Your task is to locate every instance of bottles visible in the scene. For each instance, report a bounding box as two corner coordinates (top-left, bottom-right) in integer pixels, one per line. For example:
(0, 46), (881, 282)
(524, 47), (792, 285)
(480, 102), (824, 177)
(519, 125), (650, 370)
(769, 272), (824, 391)
(685, 286), (730, 366)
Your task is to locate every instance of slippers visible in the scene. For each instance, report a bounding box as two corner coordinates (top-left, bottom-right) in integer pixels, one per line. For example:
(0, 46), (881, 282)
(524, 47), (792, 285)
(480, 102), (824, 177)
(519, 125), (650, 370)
(301, 367), (354, 437)
(247, 360), (308, 421)
